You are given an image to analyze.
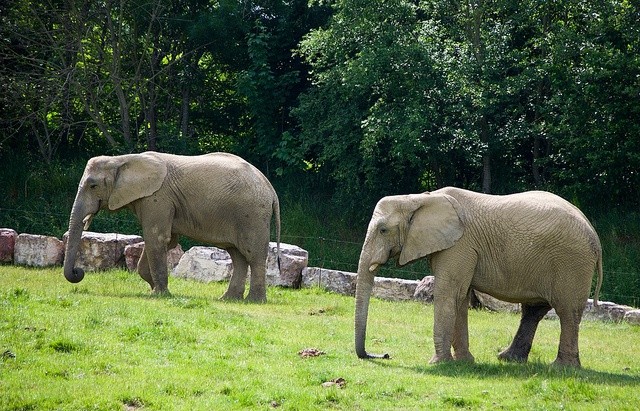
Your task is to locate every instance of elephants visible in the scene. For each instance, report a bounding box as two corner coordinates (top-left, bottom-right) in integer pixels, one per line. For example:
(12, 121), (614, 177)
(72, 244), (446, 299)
(64, 150), (282, 304)
(355, 185), (603, 369)
